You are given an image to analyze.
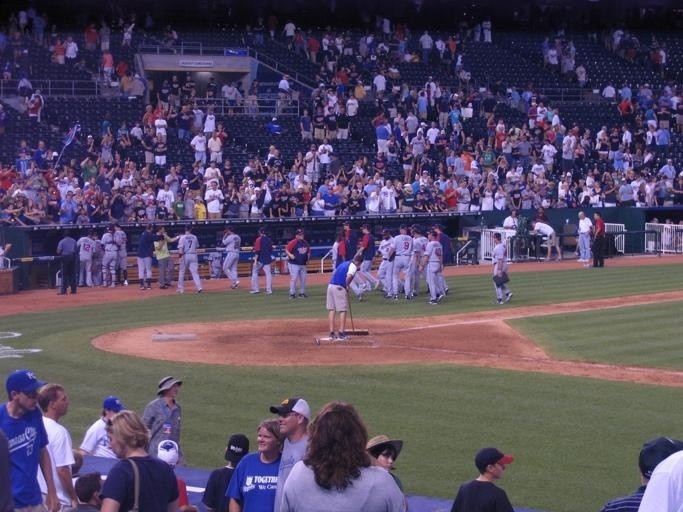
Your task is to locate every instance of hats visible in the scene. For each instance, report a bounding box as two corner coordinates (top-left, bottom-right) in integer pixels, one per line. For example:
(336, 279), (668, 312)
(493, 232), (503, 241)
(366, 434), (402, 458)
(156, 376), (182, 396)
(103, 397), (125, 414)
(158, 440), (179, 465)
(7, 370), (48, 392)
(639, 437), (683, 478)
(225, 434), (250, 461)
(475, 448), (514, 466)
(270, 397), (312, 421)
(63, 224), (444, 240)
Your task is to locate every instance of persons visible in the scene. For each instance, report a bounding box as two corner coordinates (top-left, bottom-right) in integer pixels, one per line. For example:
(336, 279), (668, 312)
(268, 396), (312, 512)
(365, 435), (405, 492)
(0, 0), (682, 305)
(449, 446), (514, 512)
(222, 418), (287, 512)
(200, 432), (251, 512)
(0, 369), (199, 511)
(636, 449), (683, 512)
(600, 436), (681, 512)
(324, 254), (364, 340)
(279, 400), (408, 512)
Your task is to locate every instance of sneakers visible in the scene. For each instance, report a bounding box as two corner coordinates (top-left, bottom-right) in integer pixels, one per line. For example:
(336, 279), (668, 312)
(505, 292), (513, 302)
(59, 282), (306, 299)
(337, 332), (350, 339)
(497, 299), (504, 304)
(357, 280), (448, 304)
(329, 331), (335, 340)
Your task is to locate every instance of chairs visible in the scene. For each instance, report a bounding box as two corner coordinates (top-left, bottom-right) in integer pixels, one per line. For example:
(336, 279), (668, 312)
(0, 28), (683, 188)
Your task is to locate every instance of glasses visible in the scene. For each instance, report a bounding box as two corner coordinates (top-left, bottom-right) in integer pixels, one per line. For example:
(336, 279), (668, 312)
(279, 414), (298, 418)
